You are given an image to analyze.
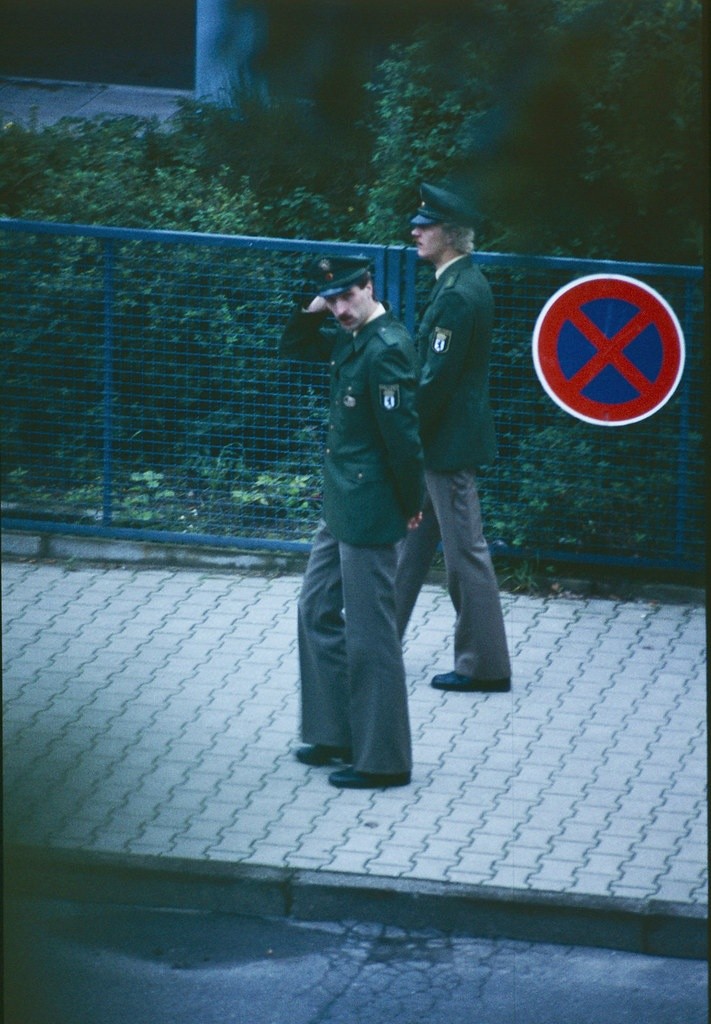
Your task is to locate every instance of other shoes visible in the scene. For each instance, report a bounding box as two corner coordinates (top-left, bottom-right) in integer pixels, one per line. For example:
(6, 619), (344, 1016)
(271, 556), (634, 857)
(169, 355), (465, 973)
(431, 671), (511, 692)
(296, 744), (353, 765)
(328, 766), (412, 788)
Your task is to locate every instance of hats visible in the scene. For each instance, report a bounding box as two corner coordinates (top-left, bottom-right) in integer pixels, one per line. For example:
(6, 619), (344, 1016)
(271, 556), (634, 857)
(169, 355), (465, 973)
(410, 183), (487, 225)
(307, 254), (376, 298)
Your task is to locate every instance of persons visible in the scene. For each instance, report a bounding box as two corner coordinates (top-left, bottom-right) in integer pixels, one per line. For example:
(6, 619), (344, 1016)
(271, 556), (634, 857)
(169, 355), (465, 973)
(398, 184), (513, 694)
(282, 257), (427, 790)
